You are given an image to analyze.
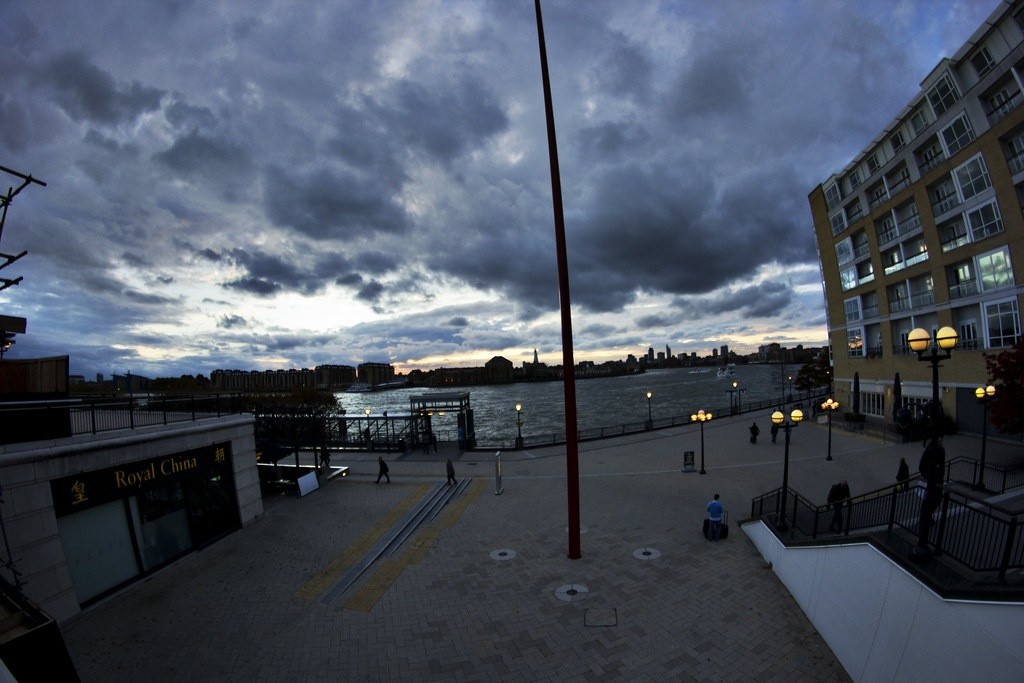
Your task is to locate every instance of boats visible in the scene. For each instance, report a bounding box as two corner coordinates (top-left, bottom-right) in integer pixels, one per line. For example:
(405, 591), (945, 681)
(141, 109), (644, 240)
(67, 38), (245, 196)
(717, 365), (736, 377)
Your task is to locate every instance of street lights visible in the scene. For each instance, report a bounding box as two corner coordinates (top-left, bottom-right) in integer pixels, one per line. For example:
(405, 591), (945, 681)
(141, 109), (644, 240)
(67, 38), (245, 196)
(821, 398), (840, 461)
(732, 382), (738, 407)
(516, 403), (524, 448)
(771, 410), (804, 532)
(691, 409), (713, 474)
(364, 408), (374, 451)
(788, 376), (792, 397)
(908, 326), (960, 449)
(646, 391), (654, 429)
(975, 385), (996, 489)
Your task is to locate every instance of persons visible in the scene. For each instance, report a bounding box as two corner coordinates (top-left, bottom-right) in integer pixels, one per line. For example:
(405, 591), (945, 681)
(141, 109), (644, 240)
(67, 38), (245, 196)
(828, 480), (851, 508)
(706, 494), (723, 543)
(750, 423), (760, 445)
(423, 433), (429, 455)
(432, 433), (437, 453)
(771, 423), (778, 444)
(319, 445), (330, 466)
(446, 459), (457, 485)
(374, 456), (391, 484)
(897, 457), (910, 482)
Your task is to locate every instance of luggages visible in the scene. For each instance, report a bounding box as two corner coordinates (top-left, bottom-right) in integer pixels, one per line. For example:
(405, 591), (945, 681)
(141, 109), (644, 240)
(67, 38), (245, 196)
(720, 511), (729, 539)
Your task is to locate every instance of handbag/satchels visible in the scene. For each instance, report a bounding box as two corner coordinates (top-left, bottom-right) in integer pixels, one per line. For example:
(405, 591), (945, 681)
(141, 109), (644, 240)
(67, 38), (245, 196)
(750, 436), (753, 442)
(703, 518), (711, 539)
